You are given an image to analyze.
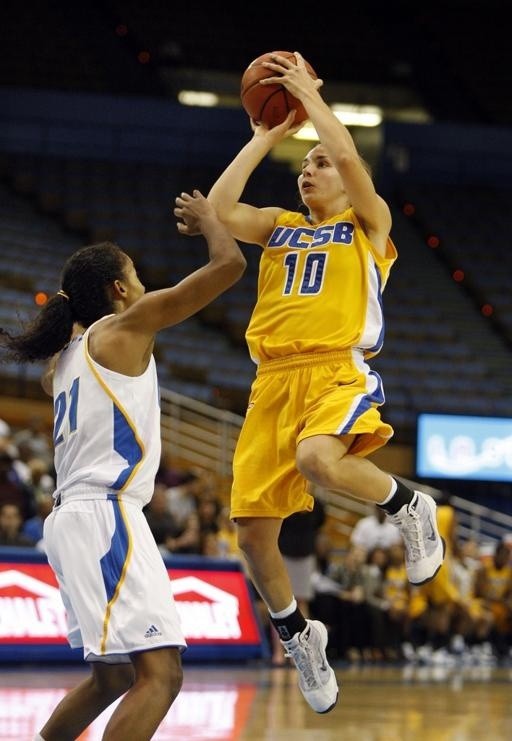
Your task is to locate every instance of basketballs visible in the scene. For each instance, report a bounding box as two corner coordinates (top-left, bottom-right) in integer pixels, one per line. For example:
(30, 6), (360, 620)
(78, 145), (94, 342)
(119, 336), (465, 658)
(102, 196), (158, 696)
(240, 51), (321, 127)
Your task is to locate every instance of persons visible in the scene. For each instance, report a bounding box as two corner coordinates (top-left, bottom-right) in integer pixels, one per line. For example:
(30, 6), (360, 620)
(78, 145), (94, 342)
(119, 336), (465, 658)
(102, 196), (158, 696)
(204, 52), (447, 715)
(11, 188), (253, 737)
(1, 417), (512, 664)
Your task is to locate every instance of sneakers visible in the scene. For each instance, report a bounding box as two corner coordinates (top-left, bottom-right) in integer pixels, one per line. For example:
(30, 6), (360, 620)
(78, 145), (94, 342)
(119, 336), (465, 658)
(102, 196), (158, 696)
(279, 618), (339, 714)
(385, 485), (446, 586)
